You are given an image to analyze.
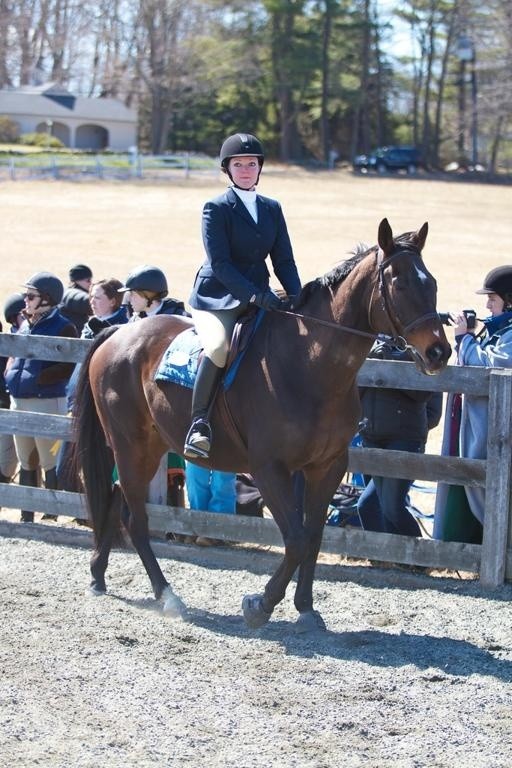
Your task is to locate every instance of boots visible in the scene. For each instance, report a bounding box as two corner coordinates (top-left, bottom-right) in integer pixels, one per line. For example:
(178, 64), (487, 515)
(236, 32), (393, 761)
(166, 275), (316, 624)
(19, 467), (37, 522)
(183, 356), (225, 458)
(40, 467), (57, 521)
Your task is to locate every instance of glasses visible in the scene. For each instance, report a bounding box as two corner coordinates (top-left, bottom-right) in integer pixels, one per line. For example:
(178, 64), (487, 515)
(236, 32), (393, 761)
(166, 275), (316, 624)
(22, 293), (41, 300)
(84, 279), (91, 283)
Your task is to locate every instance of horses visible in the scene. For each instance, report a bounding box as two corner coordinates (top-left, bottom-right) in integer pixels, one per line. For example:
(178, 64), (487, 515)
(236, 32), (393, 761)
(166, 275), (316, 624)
(65, 216), (453, 635)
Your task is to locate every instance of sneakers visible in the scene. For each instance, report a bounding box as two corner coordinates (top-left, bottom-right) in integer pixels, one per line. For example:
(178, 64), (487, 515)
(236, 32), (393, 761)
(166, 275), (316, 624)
(196, 536), (223, 547)
(183, 536), (196, 545)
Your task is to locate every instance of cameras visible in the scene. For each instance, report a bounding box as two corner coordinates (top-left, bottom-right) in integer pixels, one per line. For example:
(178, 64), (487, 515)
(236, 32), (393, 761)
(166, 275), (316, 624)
(438, 310), (477, 330)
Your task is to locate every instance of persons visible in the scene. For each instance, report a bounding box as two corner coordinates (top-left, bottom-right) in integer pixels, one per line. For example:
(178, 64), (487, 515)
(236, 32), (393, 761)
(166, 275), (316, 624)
(0, 265), (237, 545)
(358, 330), (435, 539)
(180, 131), (303, 460)
(454, 265), (512, 526)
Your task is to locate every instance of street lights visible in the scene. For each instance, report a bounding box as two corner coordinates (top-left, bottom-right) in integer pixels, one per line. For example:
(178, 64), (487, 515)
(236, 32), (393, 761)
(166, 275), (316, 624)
(457, 16), (478, 171)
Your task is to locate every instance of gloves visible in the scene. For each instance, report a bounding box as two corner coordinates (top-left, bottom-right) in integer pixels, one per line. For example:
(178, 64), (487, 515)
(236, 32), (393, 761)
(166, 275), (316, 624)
(253, 290), (281, 312)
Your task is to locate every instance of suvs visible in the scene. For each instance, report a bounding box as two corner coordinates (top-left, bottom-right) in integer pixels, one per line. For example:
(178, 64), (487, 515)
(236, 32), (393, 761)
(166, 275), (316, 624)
(351, 146), (421, 175)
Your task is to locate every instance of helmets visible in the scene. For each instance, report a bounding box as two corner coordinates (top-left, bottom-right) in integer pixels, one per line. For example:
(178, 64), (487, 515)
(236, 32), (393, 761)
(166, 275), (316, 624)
(4, 294), (26, 322)
(117, 267), (167, 293)
(476, 265), (512, 296)
(219, 133), (264, 167)
(25, 271), (64, 303)
(69, 264), (92, 281)
